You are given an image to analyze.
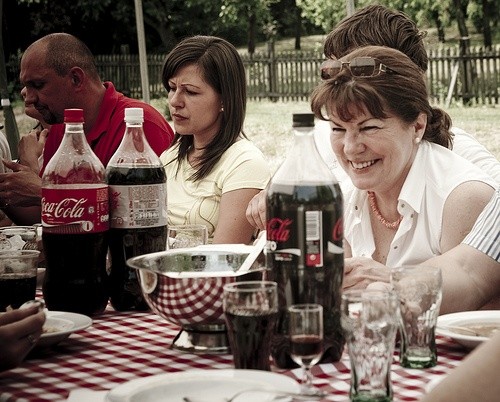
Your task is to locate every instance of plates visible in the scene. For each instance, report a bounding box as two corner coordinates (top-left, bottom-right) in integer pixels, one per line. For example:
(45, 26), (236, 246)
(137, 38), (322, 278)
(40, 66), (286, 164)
(102, 370), (304, 401)
(0, 311), (94, 347)
(434, 310), (500, 347)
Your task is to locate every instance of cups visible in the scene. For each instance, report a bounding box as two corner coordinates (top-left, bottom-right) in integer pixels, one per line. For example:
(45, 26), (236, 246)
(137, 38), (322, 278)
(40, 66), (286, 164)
(341, 289), (400, 402)
(390, 265), (443, 370)
(222, 280), (278, 372)
(0, 250), (41, 313)
(0, 226), (38, 251)
(167, 225), (208, 251)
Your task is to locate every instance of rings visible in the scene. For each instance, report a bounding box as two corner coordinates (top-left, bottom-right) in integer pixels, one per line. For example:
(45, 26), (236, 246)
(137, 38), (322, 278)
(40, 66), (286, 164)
(29, 335), (39, 347)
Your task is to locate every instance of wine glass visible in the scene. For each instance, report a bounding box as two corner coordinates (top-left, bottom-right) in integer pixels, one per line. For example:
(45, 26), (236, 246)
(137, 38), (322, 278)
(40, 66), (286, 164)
(288, 304), (328, 396)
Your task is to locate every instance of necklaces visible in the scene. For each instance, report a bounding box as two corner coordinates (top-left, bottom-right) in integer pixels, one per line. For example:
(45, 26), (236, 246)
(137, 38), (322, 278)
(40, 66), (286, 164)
(370, 190), (403, 229)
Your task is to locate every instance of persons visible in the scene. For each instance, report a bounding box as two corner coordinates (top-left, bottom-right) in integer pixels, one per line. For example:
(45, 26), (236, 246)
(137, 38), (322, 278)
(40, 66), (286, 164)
(311, 7), (500, 214)
(157, 36), (271, 244)
(342, 186), (500, 314)
(417, 324), (500, 401)
(0, 302), (46, 372)
(0, 33), (175, 226)
(245, 45), (497, 302)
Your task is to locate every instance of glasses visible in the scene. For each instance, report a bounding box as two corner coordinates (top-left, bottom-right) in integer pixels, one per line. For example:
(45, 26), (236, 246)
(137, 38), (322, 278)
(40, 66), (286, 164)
(318, 56), (406, 79)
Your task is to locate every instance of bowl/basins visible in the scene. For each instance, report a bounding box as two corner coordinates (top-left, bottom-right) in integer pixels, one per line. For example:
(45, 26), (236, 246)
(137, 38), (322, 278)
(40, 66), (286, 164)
(124, 243), (271, 350)
(37, 268), (46, 287)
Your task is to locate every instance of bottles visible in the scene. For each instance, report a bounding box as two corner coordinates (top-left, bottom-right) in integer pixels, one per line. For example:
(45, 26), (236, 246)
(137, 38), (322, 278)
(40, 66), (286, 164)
(262, 112), (344, 366)
(106, 107), (167, 313)
(41, 108), (110, 316)
